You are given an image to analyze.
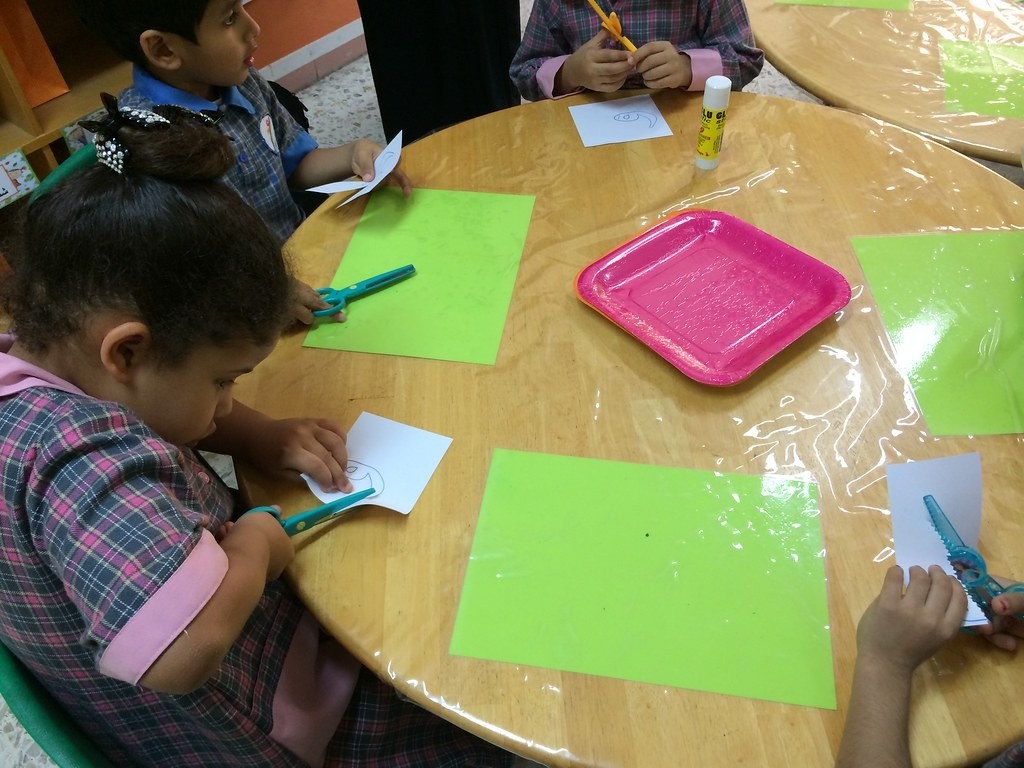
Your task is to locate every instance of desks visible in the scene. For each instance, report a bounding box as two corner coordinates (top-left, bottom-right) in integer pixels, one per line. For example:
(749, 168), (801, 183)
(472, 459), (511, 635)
(744, 0), (1024, 168)
(232, 88), (1024, 768)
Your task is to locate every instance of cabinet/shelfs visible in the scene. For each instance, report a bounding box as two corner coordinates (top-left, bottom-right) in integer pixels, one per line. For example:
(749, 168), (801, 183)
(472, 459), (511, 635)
(0, 1), (132, 335)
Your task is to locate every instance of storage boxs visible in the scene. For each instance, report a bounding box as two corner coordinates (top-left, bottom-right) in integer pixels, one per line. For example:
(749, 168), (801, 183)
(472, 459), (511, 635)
(0, 0), (70, 110)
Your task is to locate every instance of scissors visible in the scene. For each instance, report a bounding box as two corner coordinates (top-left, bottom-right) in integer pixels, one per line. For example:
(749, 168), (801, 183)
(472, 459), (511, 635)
(589, 0), (637, 52)
(309, 263), (416, 318)
(921, 492), (1024, 639)
(240, 487), (375, 536)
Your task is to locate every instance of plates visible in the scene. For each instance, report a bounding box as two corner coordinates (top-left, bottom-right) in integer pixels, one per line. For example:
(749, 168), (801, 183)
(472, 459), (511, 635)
(572, 207), (853, 386)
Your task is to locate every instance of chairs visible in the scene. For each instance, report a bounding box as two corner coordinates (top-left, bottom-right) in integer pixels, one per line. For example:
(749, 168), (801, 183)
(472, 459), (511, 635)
(0, 636), (117, 768)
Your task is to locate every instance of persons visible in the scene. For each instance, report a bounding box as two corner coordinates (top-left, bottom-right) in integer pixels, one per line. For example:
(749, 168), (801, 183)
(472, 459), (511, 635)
(0, 91), (514, 767)
(509, 0), (765, 101)
(834, 563), (1024, 768)
(116, 1), (413, 325)
